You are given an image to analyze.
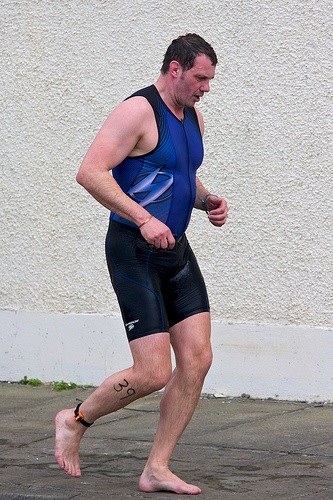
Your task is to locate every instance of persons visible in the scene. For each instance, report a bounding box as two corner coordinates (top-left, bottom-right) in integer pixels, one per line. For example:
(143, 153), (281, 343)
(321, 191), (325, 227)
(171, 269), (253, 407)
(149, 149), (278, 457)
(53, 33), (231, 494)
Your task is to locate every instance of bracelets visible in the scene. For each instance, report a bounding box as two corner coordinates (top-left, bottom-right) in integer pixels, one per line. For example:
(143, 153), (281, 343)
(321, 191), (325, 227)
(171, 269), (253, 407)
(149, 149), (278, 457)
(202, 195), (219, 214)
(138, 214), (153, 229)
